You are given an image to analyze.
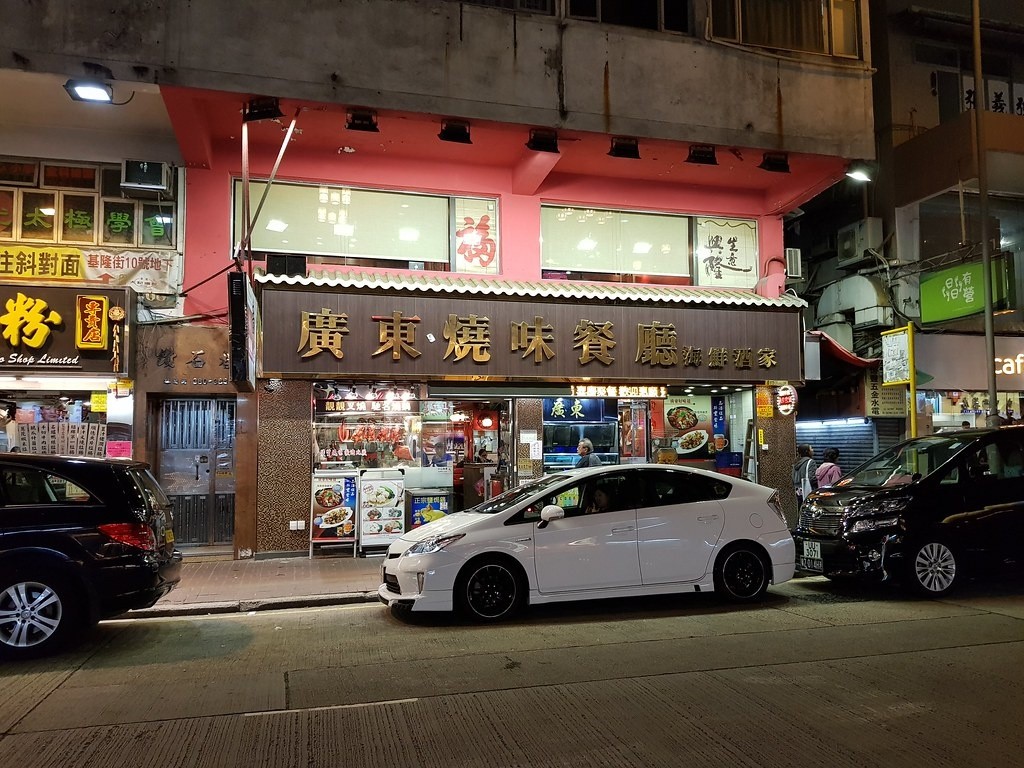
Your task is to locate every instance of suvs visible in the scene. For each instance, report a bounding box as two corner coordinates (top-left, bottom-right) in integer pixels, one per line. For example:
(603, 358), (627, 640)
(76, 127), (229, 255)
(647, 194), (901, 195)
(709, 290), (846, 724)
(0, 452), (184, 663)
(789, 424), (1024, 602)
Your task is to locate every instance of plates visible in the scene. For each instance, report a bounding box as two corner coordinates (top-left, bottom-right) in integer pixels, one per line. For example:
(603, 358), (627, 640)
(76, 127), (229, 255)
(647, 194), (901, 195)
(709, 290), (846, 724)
(667, 406), (698, 430)
(676, 429), (708, 454)
(318, 507), (353, 528)
(361, 483), (403, 534)
(315, 488), (343, 508)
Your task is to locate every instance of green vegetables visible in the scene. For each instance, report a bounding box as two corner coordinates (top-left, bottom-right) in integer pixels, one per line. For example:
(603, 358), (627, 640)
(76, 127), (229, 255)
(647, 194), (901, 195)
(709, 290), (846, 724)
(378, 509), (402, 532)
(380, 485), (395, 499)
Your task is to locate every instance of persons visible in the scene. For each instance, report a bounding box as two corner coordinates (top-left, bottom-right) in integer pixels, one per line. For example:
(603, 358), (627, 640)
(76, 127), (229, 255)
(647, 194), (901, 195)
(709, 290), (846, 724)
(414, 442), (453, 467)
(38, 403), (64, 423)
(5, 446), (26, 486)
(476, 449), (493, 464)
(962, 421), (971, 430)
(584, 485), (612, 515)
(576, 437), (604, 468)
(793, 443), (842, 520)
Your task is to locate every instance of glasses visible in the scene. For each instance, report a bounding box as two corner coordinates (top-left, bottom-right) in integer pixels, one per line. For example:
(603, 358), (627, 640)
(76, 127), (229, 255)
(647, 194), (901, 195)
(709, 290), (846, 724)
(482, 454), (486, 457)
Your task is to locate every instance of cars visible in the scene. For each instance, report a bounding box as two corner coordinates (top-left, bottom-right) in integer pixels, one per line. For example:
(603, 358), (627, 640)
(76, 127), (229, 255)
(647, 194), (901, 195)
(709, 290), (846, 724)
(377, 463), (799, 625)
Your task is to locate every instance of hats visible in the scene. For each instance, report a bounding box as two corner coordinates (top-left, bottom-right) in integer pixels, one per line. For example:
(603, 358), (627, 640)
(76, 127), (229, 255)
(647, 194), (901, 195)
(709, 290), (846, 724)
(435, 442), (444, 447)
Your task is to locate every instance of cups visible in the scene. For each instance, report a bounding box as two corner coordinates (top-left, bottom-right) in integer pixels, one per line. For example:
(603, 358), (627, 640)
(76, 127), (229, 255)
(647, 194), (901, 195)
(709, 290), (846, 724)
(714, 434), (728, 451)
(343, 520), (355, 534)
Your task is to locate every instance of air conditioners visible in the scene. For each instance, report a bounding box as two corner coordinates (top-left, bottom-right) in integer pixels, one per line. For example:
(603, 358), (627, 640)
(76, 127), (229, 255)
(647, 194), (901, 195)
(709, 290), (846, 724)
(120, 158), (171, 194)
(785, 246), (803, 279)
(835, 217), (885, 265)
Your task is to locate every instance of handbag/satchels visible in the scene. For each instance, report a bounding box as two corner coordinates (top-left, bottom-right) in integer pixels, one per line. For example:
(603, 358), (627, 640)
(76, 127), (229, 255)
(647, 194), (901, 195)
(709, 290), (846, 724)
(801, 458), (813, 502)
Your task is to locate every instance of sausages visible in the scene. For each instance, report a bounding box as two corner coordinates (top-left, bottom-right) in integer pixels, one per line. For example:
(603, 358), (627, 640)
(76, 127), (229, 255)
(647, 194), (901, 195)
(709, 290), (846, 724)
(337, 421), (349, 443)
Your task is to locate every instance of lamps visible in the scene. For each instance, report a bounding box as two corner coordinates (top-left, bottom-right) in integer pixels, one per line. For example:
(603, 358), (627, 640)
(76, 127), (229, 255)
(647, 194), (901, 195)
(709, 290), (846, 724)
(61, 72), (116, 105)
(757, 146), (792, 174)
(244, 95), (286, 120)
(686, 145), (721, 167)
(606, 133), (641, 159)
(525, 126), (562, 153)
(437, 117), (472, 145)
(343, 107), (383, 133)
(843, 160), (873, 185)
